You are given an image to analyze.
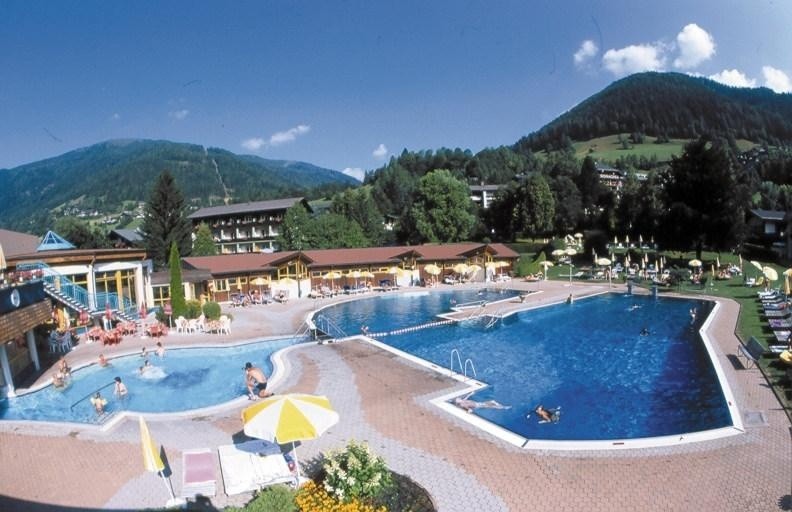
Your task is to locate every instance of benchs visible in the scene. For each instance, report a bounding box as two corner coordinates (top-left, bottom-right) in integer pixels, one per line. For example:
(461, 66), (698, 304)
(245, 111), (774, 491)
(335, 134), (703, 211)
(738, 337), (766, 369)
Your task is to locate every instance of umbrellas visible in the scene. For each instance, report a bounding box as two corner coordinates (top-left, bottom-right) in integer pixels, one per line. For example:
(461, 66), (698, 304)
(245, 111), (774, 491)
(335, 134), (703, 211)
(138, 302), (148, 338)
(79, 306), (90, 342)
(241, 393), (341, 483)
(164, 295), (174, 331)
(139, 413), (176, 499)
(106, 303), (113, 330)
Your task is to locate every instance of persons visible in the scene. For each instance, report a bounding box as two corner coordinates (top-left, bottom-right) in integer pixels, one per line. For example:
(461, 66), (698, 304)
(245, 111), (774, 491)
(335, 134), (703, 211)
(455, 391), (512, 413)
(244, 362), (274, 401)
(527, 405), (553, 424)
(53, 342), (165, 413)
(567, 293), (573, 304)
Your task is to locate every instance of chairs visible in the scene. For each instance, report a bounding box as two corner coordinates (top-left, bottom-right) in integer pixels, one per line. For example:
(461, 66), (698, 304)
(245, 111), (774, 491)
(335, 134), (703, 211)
(182, 439), (296, 498)
(175, 314), (231, 335)
(747, 278), (755, 287)
(88, 322), (168, 345)
(47, 332), (73, 352)
(757, 289), (792, 353)
(311, 287), (368, 299)
(756, 277), (764, 285)
